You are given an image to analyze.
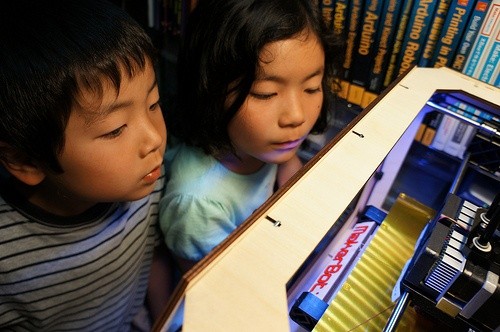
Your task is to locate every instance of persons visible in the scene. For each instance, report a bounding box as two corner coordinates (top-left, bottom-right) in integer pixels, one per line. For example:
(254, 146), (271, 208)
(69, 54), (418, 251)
(159, 0), (335, 293)
(0, 0), (174, 332)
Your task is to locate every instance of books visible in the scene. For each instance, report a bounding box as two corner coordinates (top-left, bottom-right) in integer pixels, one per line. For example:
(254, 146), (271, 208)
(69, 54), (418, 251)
(117, 0), (500, 159)
